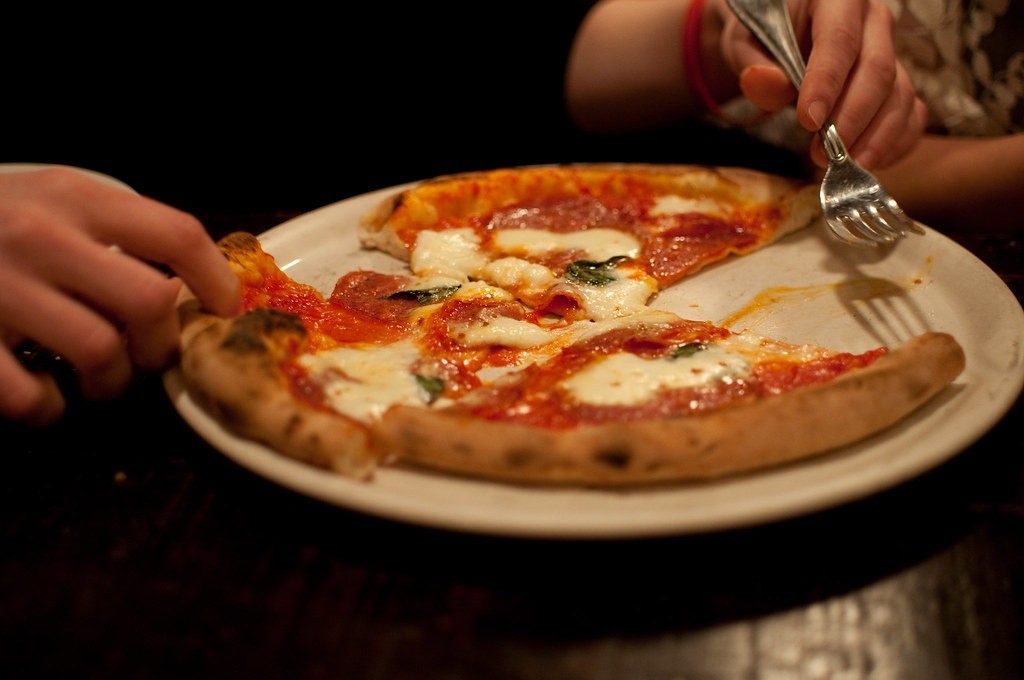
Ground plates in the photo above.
[161,180,1024,539]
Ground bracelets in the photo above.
[681,0,724,116]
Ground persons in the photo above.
[0,0,925,420]
[873,0,1024,214]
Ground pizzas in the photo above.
[356,164,825,321]
[378,306,968,484]
[176,231,543,480]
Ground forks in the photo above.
[725,0,926,250]
